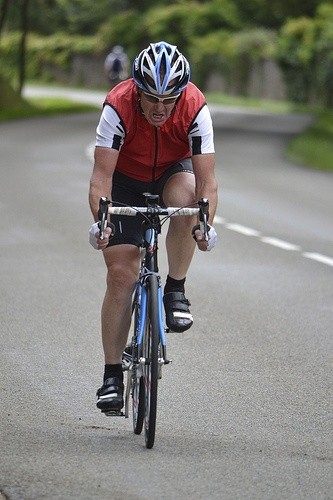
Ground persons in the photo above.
[89,41,218,413]
[105,44,131,92]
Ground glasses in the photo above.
[141,93,181,105]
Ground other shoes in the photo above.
[95,377,125,410]
[162,291,194,332]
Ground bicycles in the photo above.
[96,190,211,448]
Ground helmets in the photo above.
[132,40,191,97]
